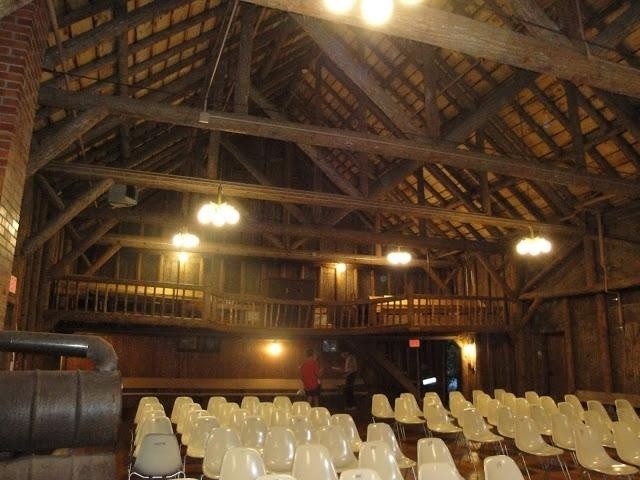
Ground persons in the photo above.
[340,349,357,395]
[298,349,321,407]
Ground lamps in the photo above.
[386,246,413,264]
[514,222,560,258]
[0,326,122,478]
[195,180,244,229]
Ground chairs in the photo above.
[131,384,640,477]
[0,326,122,478]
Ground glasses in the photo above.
[195,180,244,229]
[514,222,560,258]
[386,246,413,264]
